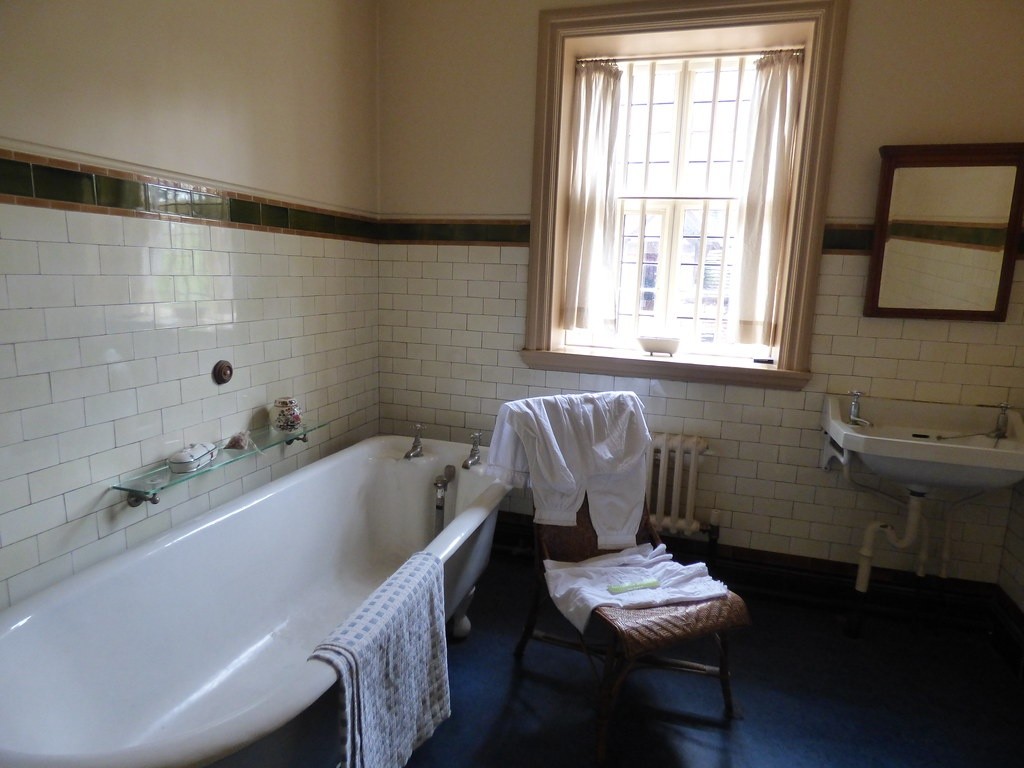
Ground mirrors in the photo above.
[863,142,1023,323]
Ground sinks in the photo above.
[819,393,1024,494]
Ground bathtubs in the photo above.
[1,434,516,768]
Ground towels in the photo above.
[305,550,452,768]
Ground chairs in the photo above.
[483,390,750,768]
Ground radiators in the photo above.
[642,431,723,558]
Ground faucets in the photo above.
[461,431,484,470]
[402,423,428,459]
[986,402,1014,440]
[848,390,874,429]
